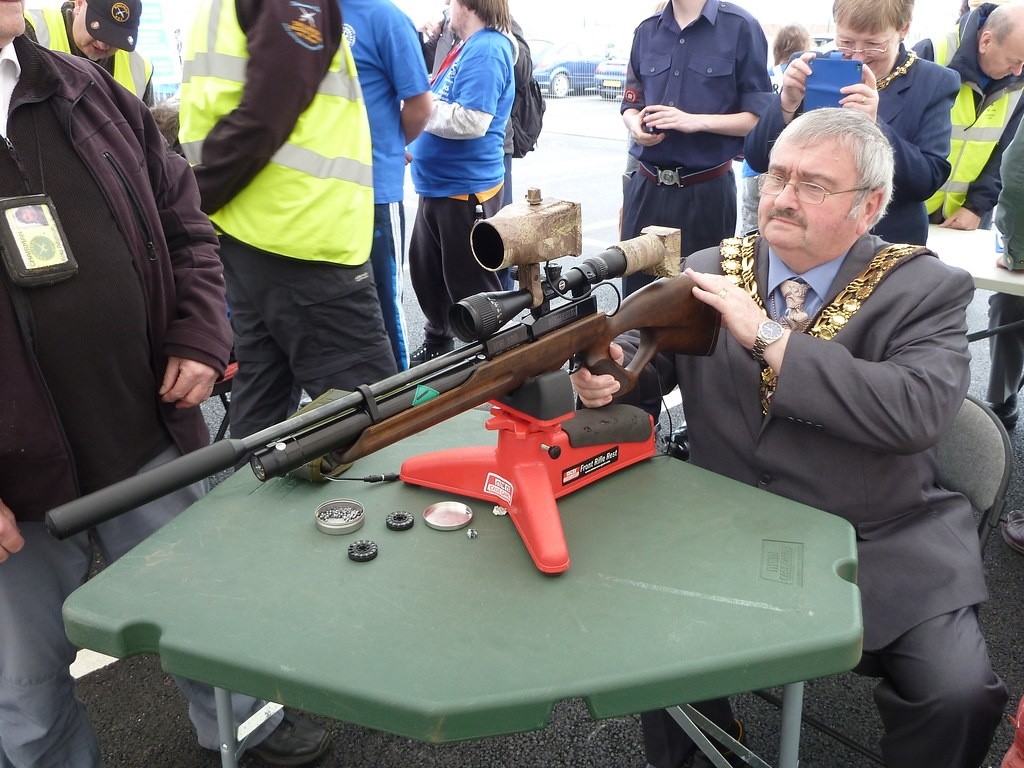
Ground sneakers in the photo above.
[409,336,455,367]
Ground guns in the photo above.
[41,187,721,543]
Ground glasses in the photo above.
[755,171,869,206]
[837,30,897,57]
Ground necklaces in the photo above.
[771,291,822,332]
[719,232,926,416]
[877,51,917,92]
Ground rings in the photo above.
[860,96,866,104]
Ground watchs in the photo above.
[752,321,785,367]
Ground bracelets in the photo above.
[781,102,801,113]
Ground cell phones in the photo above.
[804,58,863,113]
[646,112,663,133]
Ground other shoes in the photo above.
[250,710,330,766]
[1001,510,1024,554]
[664,421,689,459]
[984,392,1019,428]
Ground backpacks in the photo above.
[510,79,546,158]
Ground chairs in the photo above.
[751,393,1015,763]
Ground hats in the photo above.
[85,0,142,53]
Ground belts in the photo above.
[639,159,733,188]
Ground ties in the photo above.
[780,280,811,333]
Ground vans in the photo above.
[523,36,633,98]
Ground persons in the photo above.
[572,106,1012,768]
[741,0,961,247]
[911,1,1024,426]
[1001,508,1024,552]
[618,0,777,458]
[0,0,530,768]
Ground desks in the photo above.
[925,221,1024,343]
[61,383,863,768]
